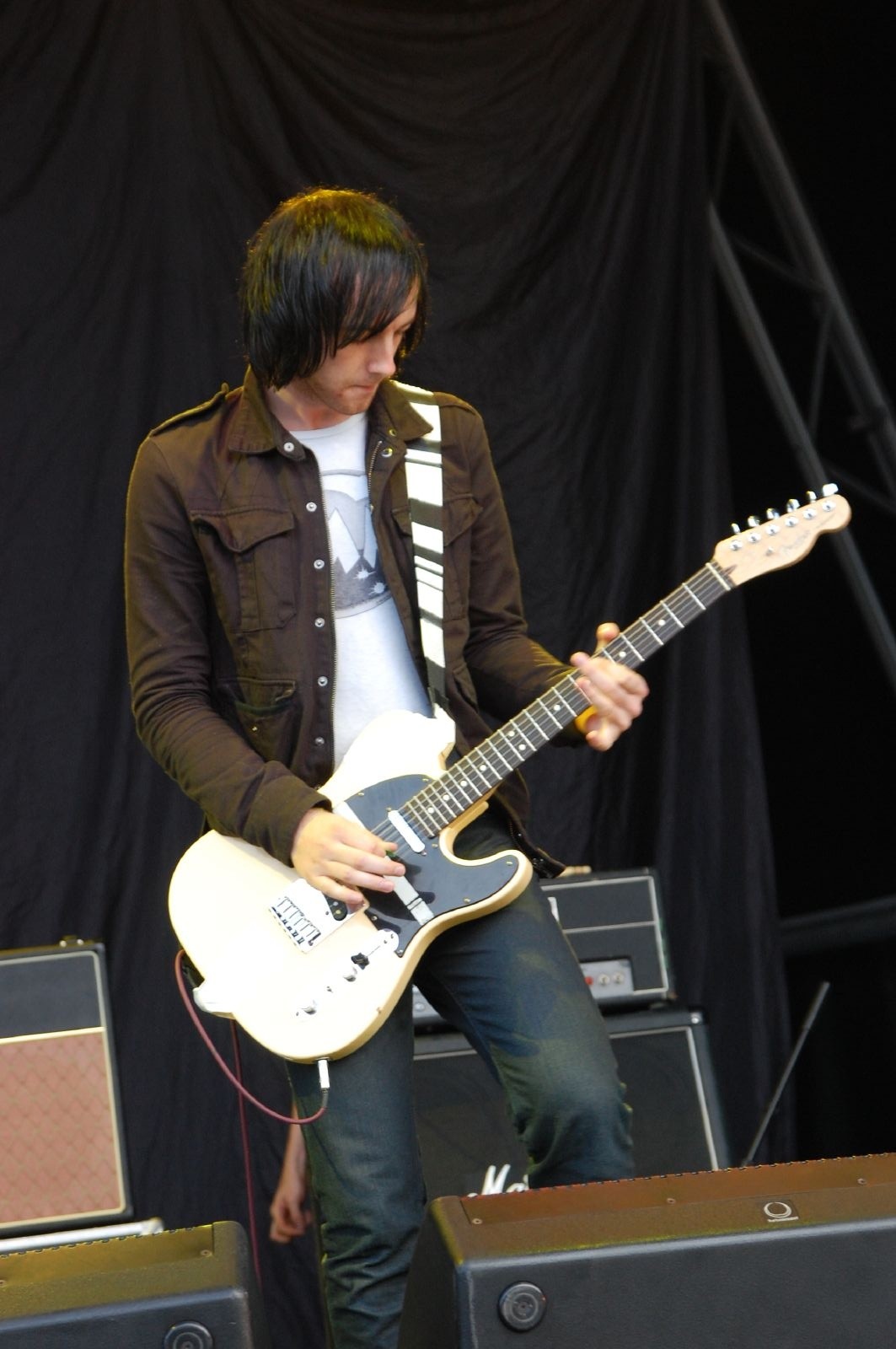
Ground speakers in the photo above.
[0,936,268,1349]
[402,864,896,1349]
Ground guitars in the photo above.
[166,481,855,1061]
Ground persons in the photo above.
[118,184,650,1349]
